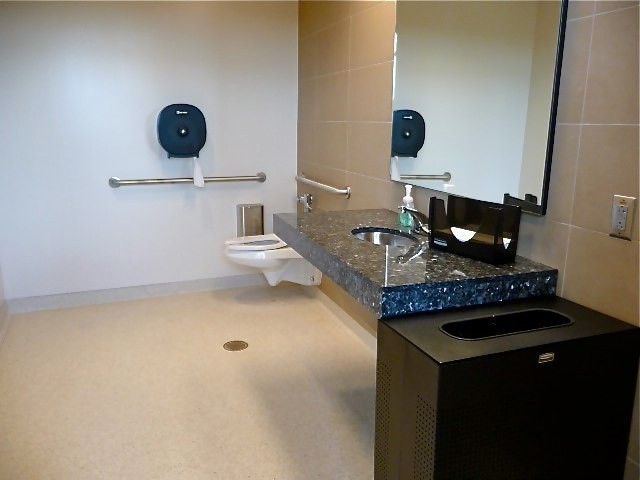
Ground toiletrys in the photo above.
[399,184,417,228]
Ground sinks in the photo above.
[351,226,417,251]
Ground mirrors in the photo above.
[389,0,569,215]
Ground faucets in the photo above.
[398,203,430,235]
[396,237,433,265]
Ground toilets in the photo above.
[222,233,323,288]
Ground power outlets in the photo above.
[609,194,637,241]
